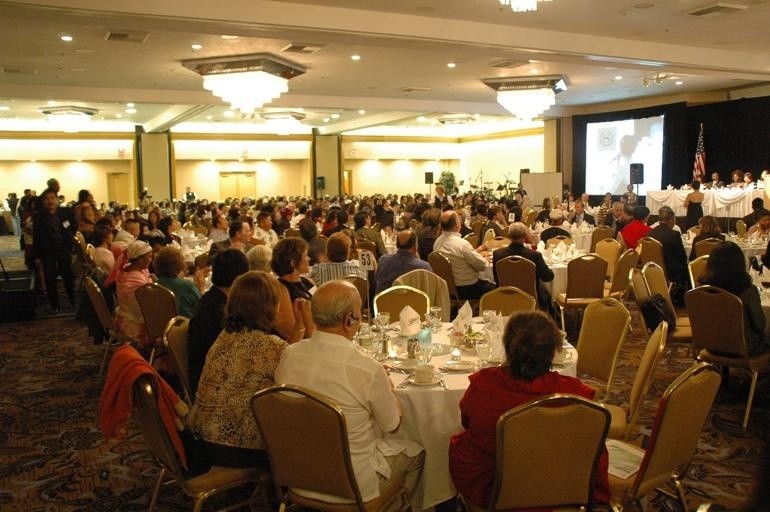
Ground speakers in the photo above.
[425,172,433,184]
[630,163,643,184]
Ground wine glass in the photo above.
[681,226,769,249]
[750,270,770,305]
[529,219,597,236]
[665,178,766,191]
[558,198,602,215]
[477,240,580,266]
[174,225,214,298]
[354,304,572,388]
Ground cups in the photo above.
[347,225,398,249]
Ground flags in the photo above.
[693,126,708,182]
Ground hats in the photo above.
[125,240,153,260]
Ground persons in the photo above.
[1,167,769,511]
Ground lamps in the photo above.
[37,105,100,134]
[180,51,307,115]
[477,74,569,123]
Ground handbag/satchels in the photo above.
[639,293,676,333]
